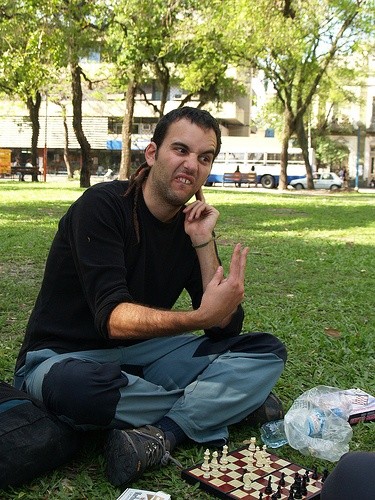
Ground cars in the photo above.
[290,172,343,191]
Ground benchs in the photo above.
[11,166,41,182]
[222,172,258,188]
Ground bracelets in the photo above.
[192,235,222,248]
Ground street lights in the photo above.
[355,120,363,192]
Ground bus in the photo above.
[204,136,315,190]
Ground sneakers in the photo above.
[96,426,183,486]
[241,392,284,424]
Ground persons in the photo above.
[234,166,242,187]
[343,167,348,187]
[247,166,256,188]
[13,106,288,487]
[96,163,104,177]
[14,156,39,183]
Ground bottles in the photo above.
[261,408,341,449]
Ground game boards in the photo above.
[181,443,324,500]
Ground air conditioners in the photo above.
[142,124,150,130]
[174,94,188,100]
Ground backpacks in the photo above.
[0,380,79,487]
